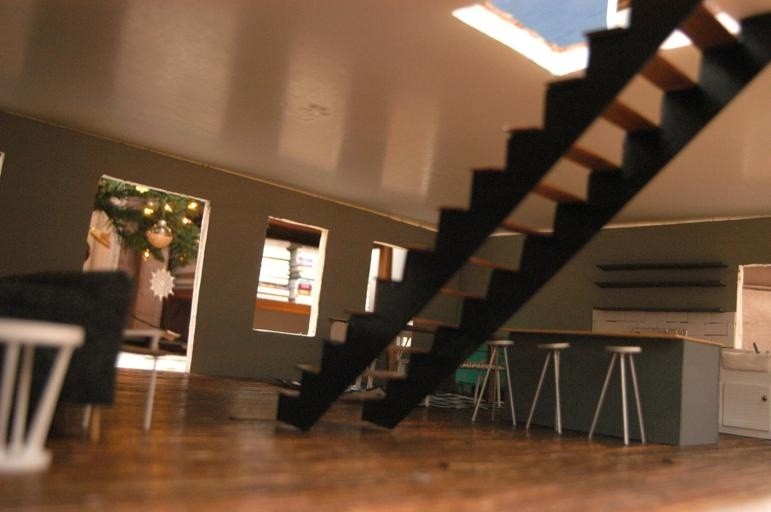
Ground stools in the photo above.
[518,343,578,434]
[585,345,649,446]
[466,338,515,424]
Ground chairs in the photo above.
[0,268,134,440]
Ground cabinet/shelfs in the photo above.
[592,256,726,314]
[719,379,769,433]
